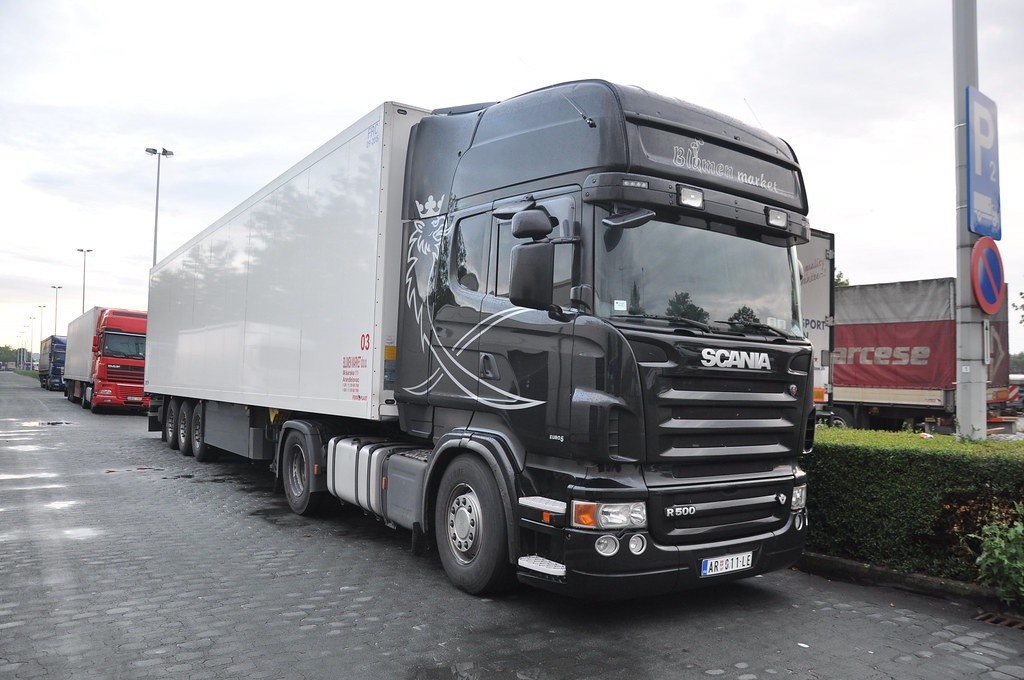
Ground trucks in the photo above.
[798,228,835,421]
[176,322,312,395]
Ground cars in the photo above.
[34,362,39,371]
[22,362,34,371]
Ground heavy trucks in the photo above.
[144,80,815,599]
[63,306,151,414]
[39,335,67,391]
[832,278,1010,436]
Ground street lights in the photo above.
[51,286,62,335]
[39,306,46,340]
[77,249,93,314]
[30,317,36,370]
[17,326,33,371]
[145,147,173,266]
